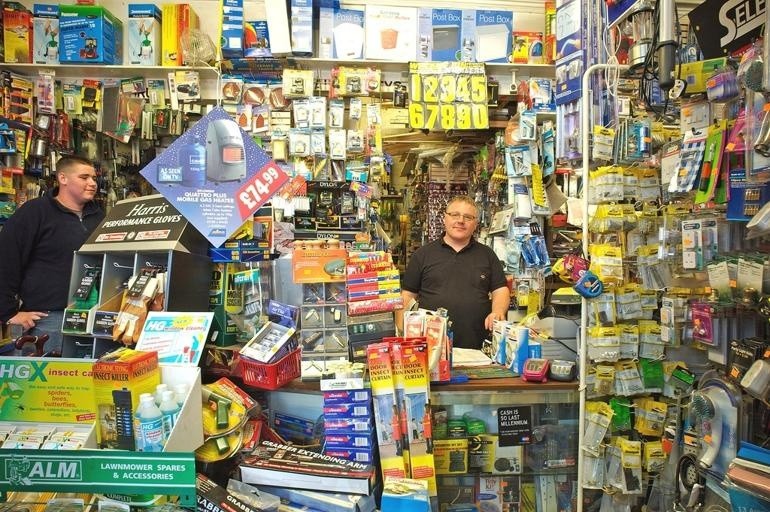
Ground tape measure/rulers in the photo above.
[573,269,604,298]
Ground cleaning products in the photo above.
[135,384,179,451]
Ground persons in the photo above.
[401,194,512,350]
[1,153,107,360]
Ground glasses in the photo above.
[446,212,475,222]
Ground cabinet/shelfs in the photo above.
[421,350,585,512]
